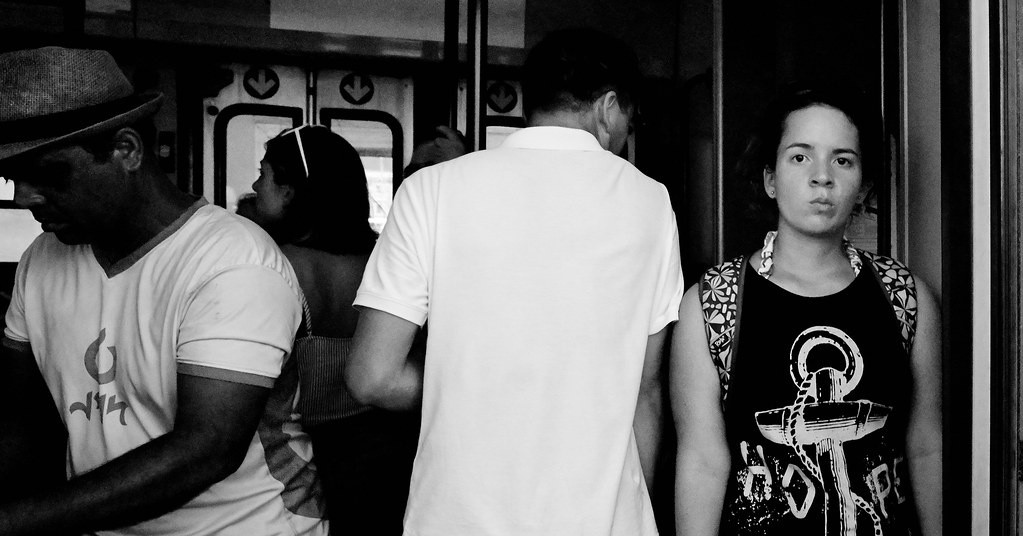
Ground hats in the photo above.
[0,46,164,164]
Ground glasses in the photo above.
[280,121,327,176]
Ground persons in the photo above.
[345,23,686,536]
[228,119,465,536]
[670,88,946,536]
[0,47,330,536]
